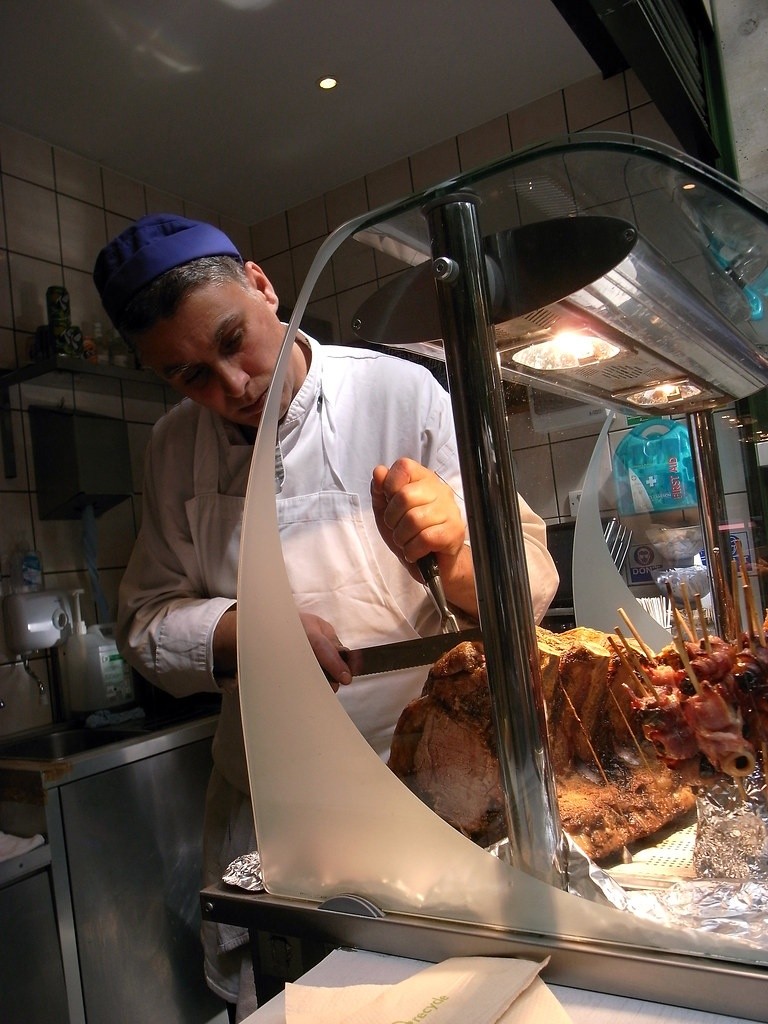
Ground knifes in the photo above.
[322,628,483,683]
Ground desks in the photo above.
[238,948,768,1024]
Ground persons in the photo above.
[92,210,558,1024]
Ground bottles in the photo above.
[92,321,109,365]
[110,328,129,368]
[87,625,135,710]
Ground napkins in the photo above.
[283,956,573,1024]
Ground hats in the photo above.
[92,214,244,326]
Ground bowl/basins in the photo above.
[645,526,703,560]
[652,565,709,604]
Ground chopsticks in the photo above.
[635,597,673,628]
